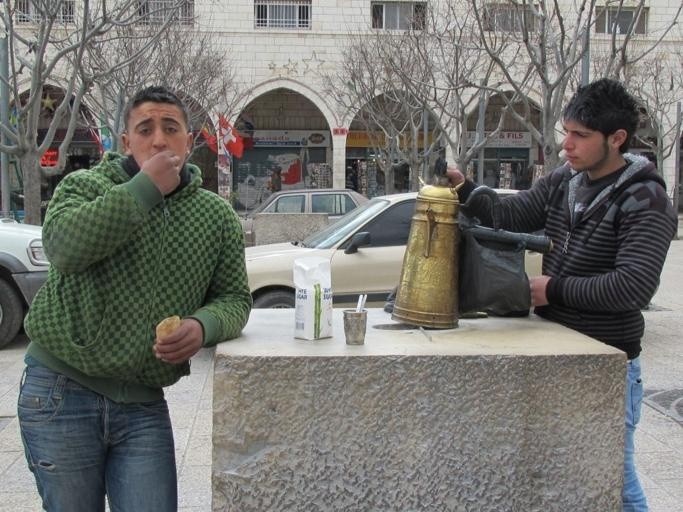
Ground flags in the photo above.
[202,127,217,153]
[98,113,112,149]
[220,114,244,159]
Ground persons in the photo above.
[18,85,254,511]
[446,77,678,512]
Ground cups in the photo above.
[343,309,368,345]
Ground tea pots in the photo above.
[391,173,461,329]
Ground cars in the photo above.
[236,186,370,246]
[0,217,51,348]
[243,189,544,309]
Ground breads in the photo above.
[156,314,180,339]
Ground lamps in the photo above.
[278,104,286,121]
